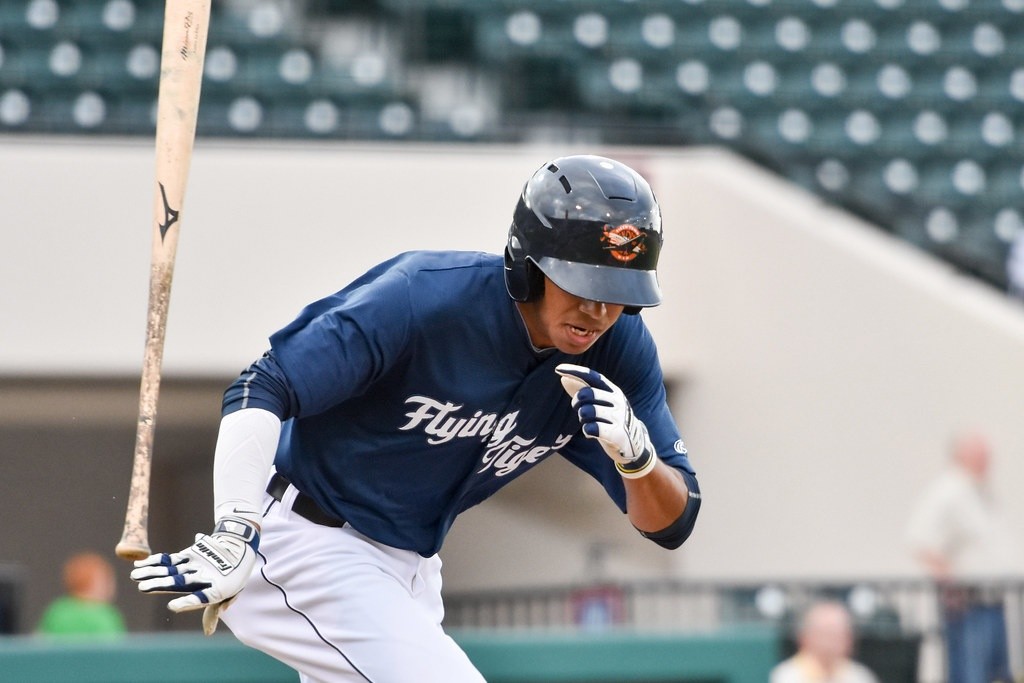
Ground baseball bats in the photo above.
[112,1,213,561]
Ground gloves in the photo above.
[130,517,259,636]
[555,363,656,479]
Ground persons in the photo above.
[565,539,628,629]
[918,426,1018,683]
[128,155,700,683]
[33,550,127,633]
[766,596,879,683]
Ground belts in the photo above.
[265,473,345,527]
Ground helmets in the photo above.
[503,155,665,317]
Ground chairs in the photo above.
[0,0,1024,288]
